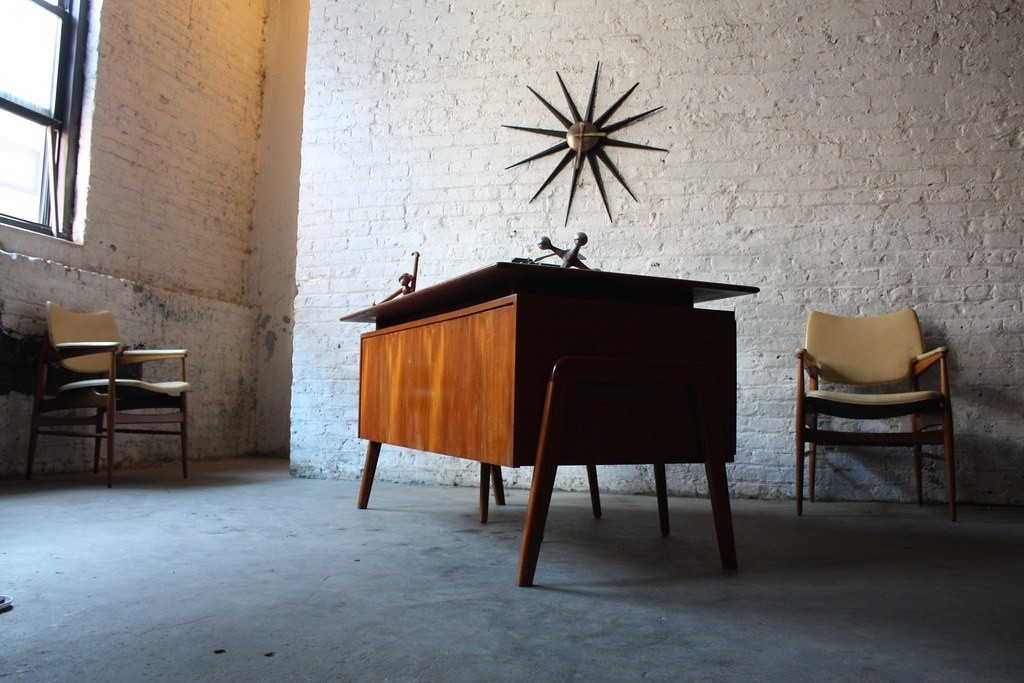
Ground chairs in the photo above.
[24,302,192,484]
[793,310,959,524]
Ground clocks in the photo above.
[499,60,669,227]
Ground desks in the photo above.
[337,262,760,586]
